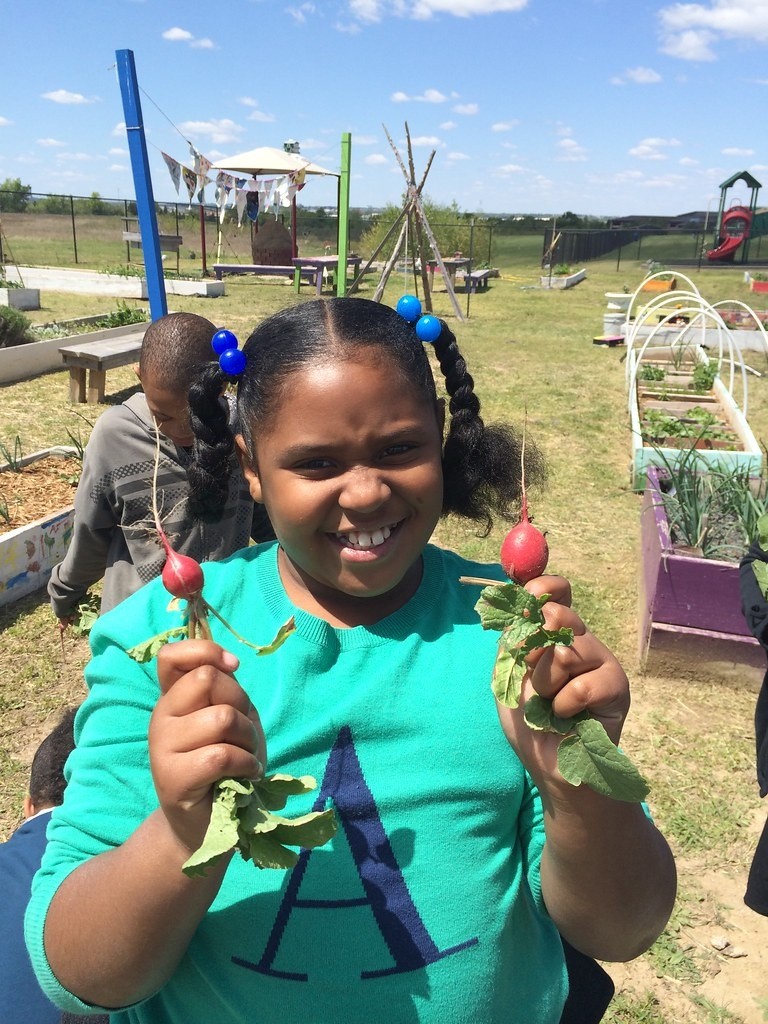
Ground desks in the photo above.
[425,257,474,293]
[291,255,364,296]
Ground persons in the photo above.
[48,311,277,627]
[0,709,76,1024]
[736,518,768,917]
[23,298,679,1024]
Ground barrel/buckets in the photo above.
[603,313,626,336]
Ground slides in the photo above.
[706,232,743,258]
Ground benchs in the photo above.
[212,262,318,288]
[463,269,490,295]
[58,322,225,406]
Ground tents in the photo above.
[207,146,340,282]
